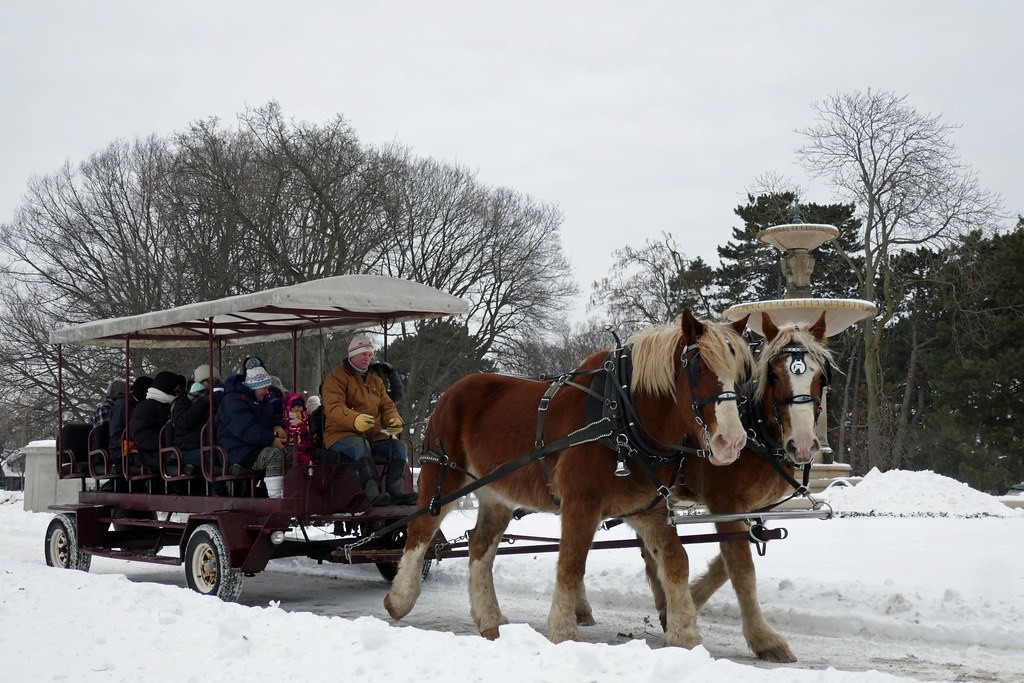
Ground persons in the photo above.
[320,332,419,507]
[90,358,288,499]
[304,394,327,468]
[287,395,321,476]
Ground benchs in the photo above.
[56,421,414,499]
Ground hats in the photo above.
[107,380,124,400]
[306,395,321,413]
[154,371,183,396]
[194,364,222,384]
[132,376,154,400]
[347,335,374,358]
[245,357,272,390]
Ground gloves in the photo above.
[387,418,403,433]
[355,414,375,432]
[382,362,392,374]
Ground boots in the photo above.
[354,457,391,507]
[386,459,418,506]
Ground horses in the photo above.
[385,308,845,663]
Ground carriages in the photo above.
[44,275,825,662]
